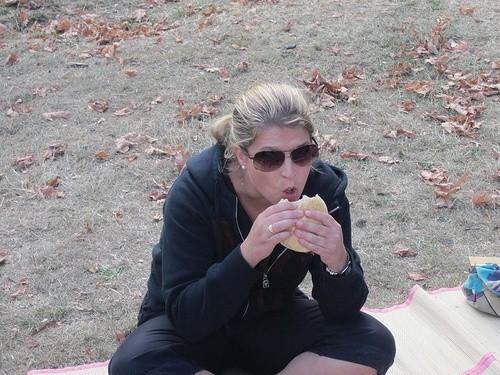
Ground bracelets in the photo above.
[326,249,351,277]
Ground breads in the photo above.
[279,195,328,253]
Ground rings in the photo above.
[269,223,275,234]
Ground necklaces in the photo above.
[238,170,268,212]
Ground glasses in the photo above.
[240,137,321,173]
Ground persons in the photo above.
[107,81,396,375]
